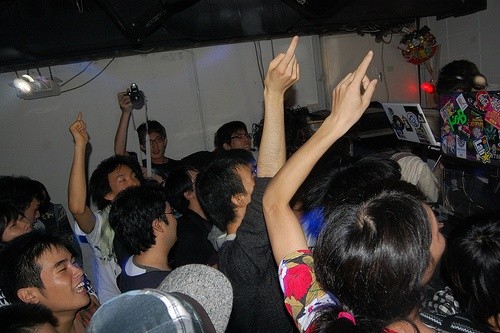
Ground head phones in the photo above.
[470,72,487,89]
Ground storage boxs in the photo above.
[351,128,393,154]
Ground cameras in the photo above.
[124,83,145,110]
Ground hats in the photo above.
[83,264,235,333]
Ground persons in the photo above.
[0,33,500,333]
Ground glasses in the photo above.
[230,133,252,140]
[150,137,164,145]
